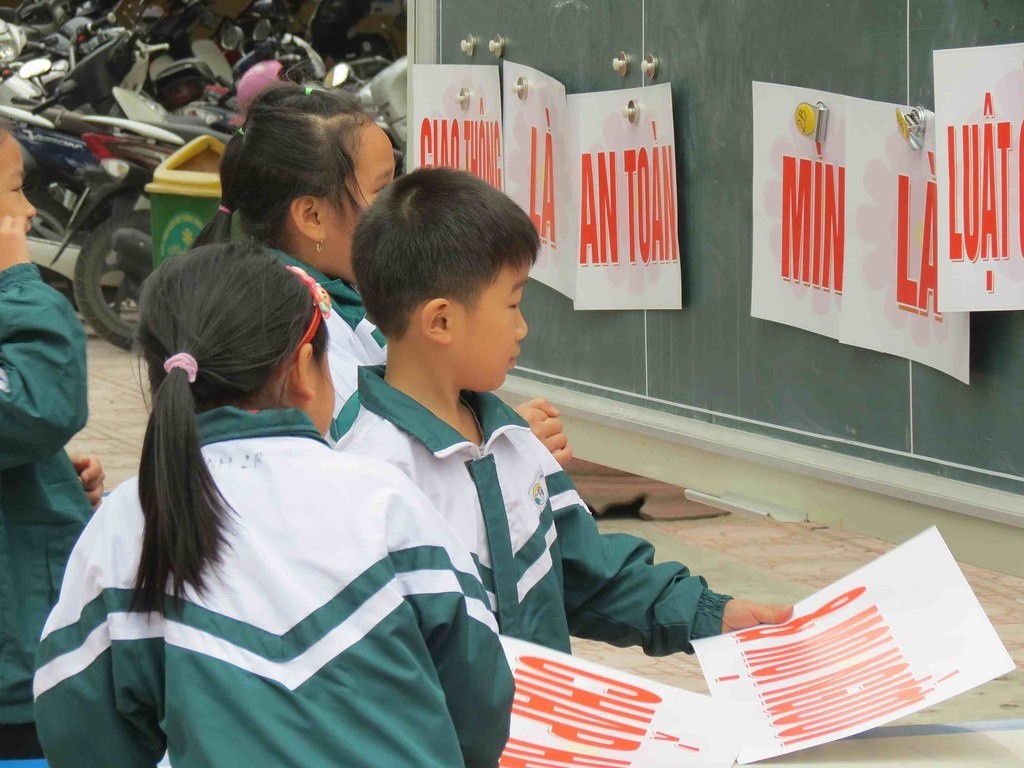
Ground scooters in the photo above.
[0,0,410,350]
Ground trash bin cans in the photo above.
[144,136,243,273]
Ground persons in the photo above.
[32,242,517,768]
[0,128,106,760]
[191,81,572,465]
[332,166,793,657]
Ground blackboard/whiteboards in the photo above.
[406,1,1024,530]
